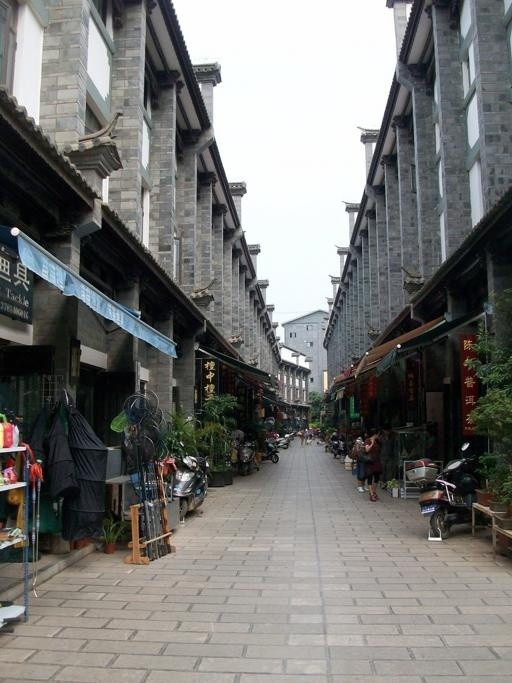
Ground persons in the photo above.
[298,426,320,446]
[351,426,393,502]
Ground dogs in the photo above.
[306,439,312,445]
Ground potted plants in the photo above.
[473,451,511,558]
[100,514,129,554]
[199,392,239,486]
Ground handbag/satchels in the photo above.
[349,444,356,459]
[359,448,374,464]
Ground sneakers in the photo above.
[371,492,379,501]
[357,486,369,492]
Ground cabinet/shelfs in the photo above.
[1,445,31,631]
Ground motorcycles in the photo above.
[166,440,211,521]
[227,429,294,476]
[405,442,487,539]
[330,432,350,459]
